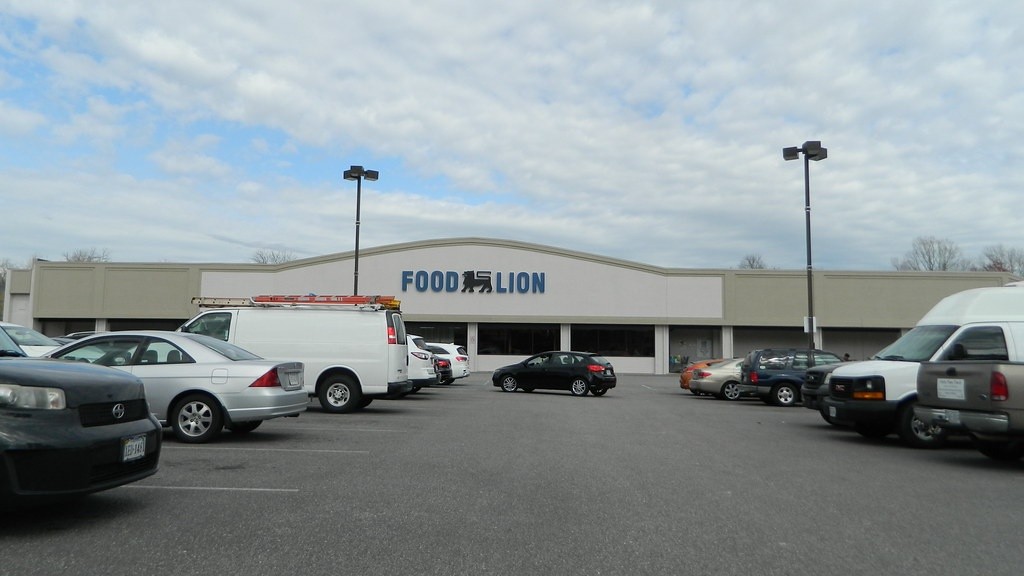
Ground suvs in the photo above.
[738,346,854,407]
[493,350,617,398]
[424,342,470,385]
[383,333,437,398]
[800,359,865,428]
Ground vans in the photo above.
[125,292,413,414]
[821,284,1023,448]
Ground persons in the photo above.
[843,353,850,360]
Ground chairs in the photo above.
[563,357,568,364]
[144,350,159,362]
[167,350,180,362]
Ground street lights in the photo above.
[783,138,830,350]
[343,164,380,296]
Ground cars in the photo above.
[36,329,310,446]
[689,357,747,402]
[407,351,455,395]
[0,321,62,357]
[50,337,105,364]
[679,360,727,395]
[0,322,164,518]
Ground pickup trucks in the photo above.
[911,358,1023,465]
